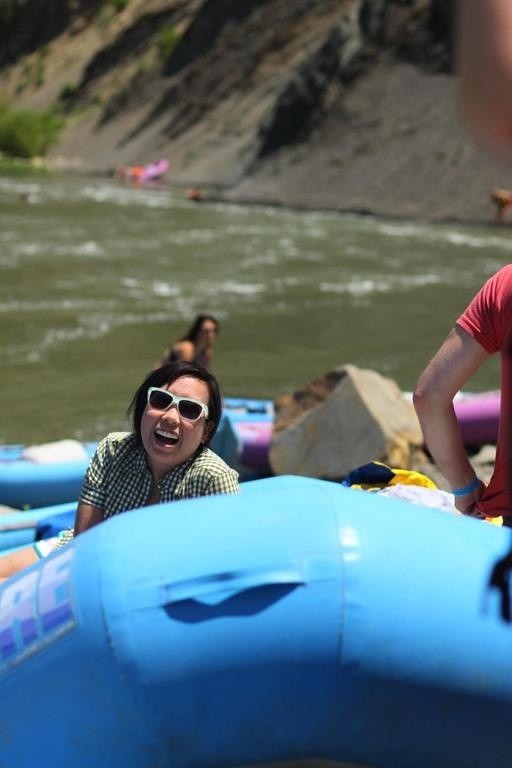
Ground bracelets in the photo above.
[447,476,481,501]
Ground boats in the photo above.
[1,398,511,768]
[127,158,169,182]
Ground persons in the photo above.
[147,311,223,378]
[1,356,242,592]
[413,262,512,534]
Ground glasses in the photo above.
[146,387,209,423]
